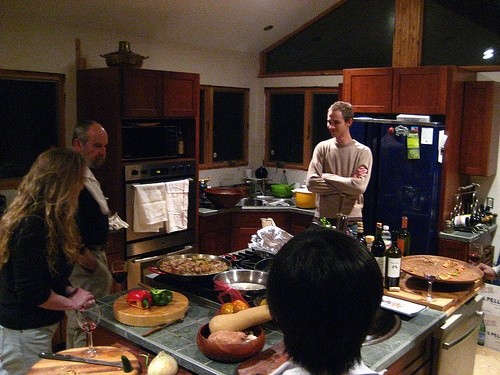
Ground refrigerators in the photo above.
[348,115,444,255]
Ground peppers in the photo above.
[121,355,132,372]
[126,288,172,310]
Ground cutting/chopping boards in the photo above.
[113,288,190,327]
[25,345,140,375]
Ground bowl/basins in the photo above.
[196,322,265,363]
[155,253,231,284]
[204,187,244,208]
[213,269,269,302]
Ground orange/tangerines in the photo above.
[220,300,268,314]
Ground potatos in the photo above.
[206,330,247,344]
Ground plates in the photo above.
[400,255,484,285]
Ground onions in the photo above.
[147,350,178,375]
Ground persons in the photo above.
[265,227,389,375]
[476,262,500,280]
[67,120,114,349]
[0,147,96,375]
[306,100,373,239]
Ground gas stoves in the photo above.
[147,247,280,331]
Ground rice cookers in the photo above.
[291,189,315,209]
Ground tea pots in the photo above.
[254,166,267,178]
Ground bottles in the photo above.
[200,181,207,200]
[245,167,252,177]
[443,220,454,234]
[300,179,306,188]
[336,216,410,289]
[467,205,493,227]
[280,170,288,184]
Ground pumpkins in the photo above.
[209,304,271,333]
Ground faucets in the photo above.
[246,178,267,196]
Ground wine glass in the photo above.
[469,242,484,266]
[76,303,101,358]
[421,264,438,303]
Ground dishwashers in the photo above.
[431,294,485,375]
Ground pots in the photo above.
[271,183,295,198]
[100,41,150,69]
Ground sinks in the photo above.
[242,196,296,208]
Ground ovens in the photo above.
[124,160,196,290]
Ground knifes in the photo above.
[38,351,123,367]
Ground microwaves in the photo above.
[121,118,196,161]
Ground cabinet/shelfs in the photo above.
[343,66,448,115]
[199,212,314,256]
[75,67,200,119]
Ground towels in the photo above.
[164,179,189,234]
[132,182,169,233]
